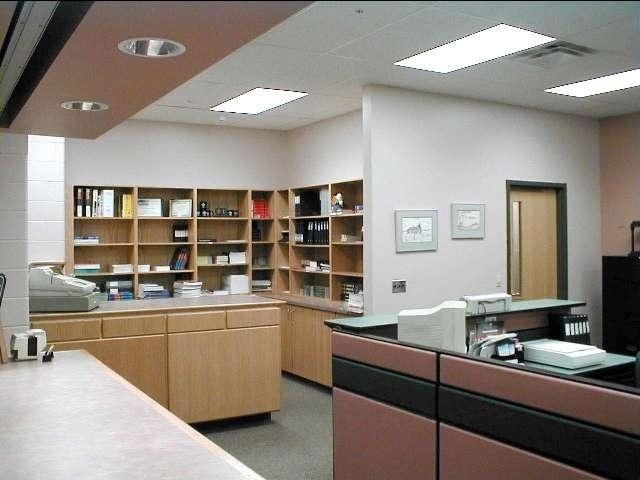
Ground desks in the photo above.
[0,349,269,480]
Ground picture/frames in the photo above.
[449,203,486,239]
[169,198,191,217]
[394,209,440,254]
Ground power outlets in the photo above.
[391,279,407,294]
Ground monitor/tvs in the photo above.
[397,301,467,354]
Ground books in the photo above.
[74,187,132,244]
[252,199,270,219]
[74,247,190,273]
[519,338,607,369]
[252,280,272,291]
[97,274,249,300]
[197,239,246,265]
[294,188,363,309]
[548,312,590,345]
[173,223,188,242]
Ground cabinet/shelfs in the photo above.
[65,182,277,297]
[277,177,363,307]
[600,253,640,355]
[166,305,280,425]
[276,300,362,388]
[31,313,169,412]
